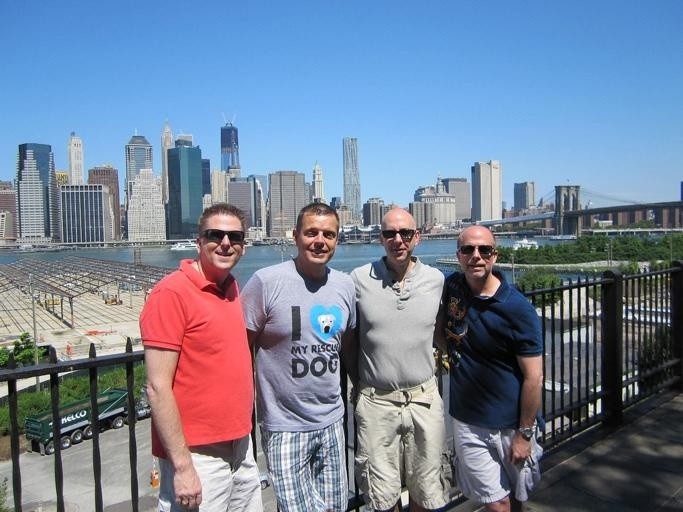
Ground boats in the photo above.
[13,244,82,252]
[170,242,198,251]
[549,234,578,240]
[511,235,539,251]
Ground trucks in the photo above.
[24,383,152,456]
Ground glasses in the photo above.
[456,245,494,257]
[199,229,247,244]
[381,229,418,241]
